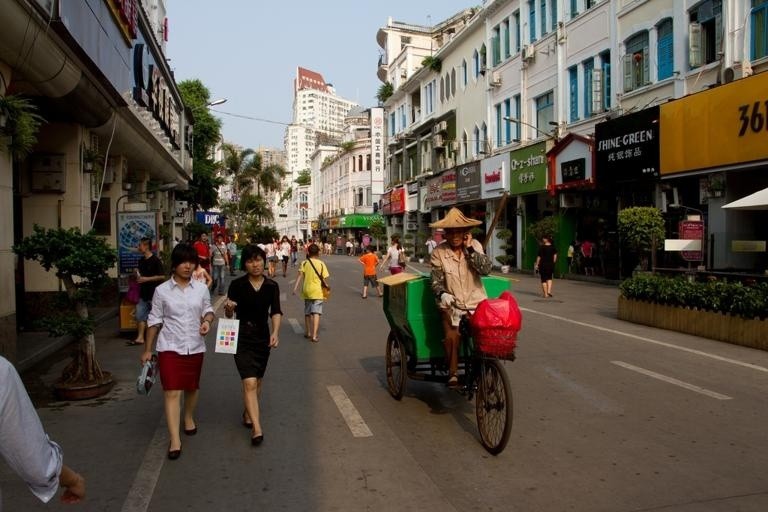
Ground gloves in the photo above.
[441,292,455,308]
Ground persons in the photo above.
[379,234,405,276]
[535,237,557,299]
[125,236,166,345]
[425,235,436,256]
[296,232,370,254]
[222,242,281,446]
[188,222,296,297]
[357,246,382,299]
[0,352,86,505]
[564,237,609,275]
[428,207,495,389]
[291,243,331,343]
[140,243,214,462]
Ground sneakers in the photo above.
[448,371,461,390]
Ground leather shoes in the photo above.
[169,436,183,459]
[184,422,196,435]
[243,408,254,429]
[251,435,263,446]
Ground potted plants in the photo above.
[402,234,414,263]
[124,176,136,189]
[0,94,49,163]
[495,230,514,274]
[146,180,162,198]
[417,232,427,263]
[10,221,120,401]
[82,146,105,171]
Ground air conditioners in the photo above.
[433,121,458,152]
[521,44,534,62]
[488,71,500,85]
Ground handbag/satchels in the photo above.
[321,285,331,302]
[136,352,159,396]
[398,251,405,265]
[127,275,140,304]
[215,317,240,355]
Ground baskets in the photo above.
[468,323,521,362]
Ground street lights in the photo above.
[192,98,228,117]
[114,182,180,288]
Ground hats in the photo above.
[428,207,483,229]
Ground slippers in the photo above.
[126,340,145,345]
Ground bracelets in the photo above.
[202,319,210,325]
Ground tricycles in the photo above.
[378,271,520,456]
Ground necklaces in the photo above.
[248,277,264,292]
